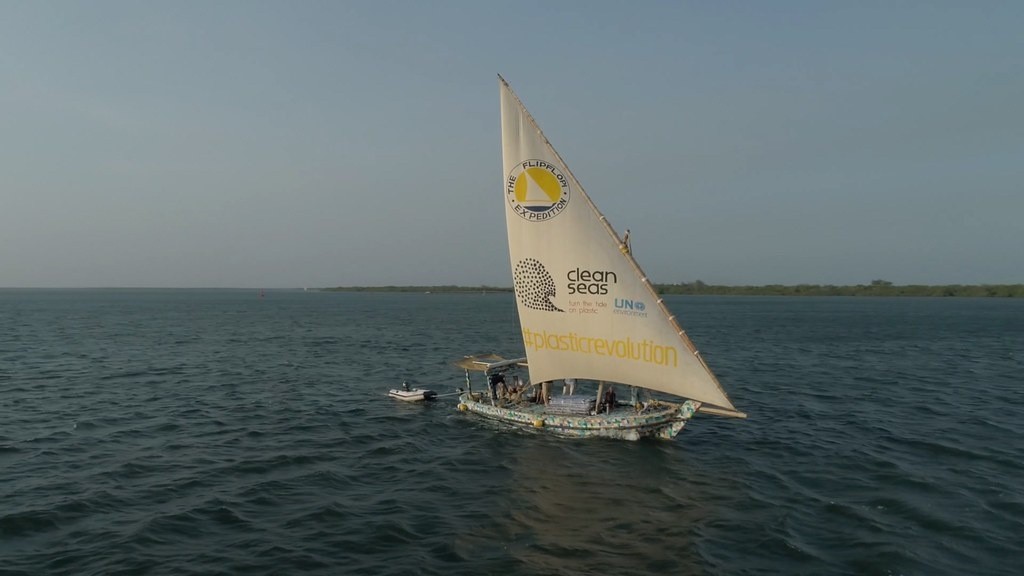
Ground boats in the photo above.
[389,380,437,402]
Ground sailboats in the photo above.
[457,72,747,441]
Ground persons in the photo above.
[629,385,641,412]
[605,386,616,411]
[561,379,578,397]
[536,380,553,404]
[491,372,509,400]
